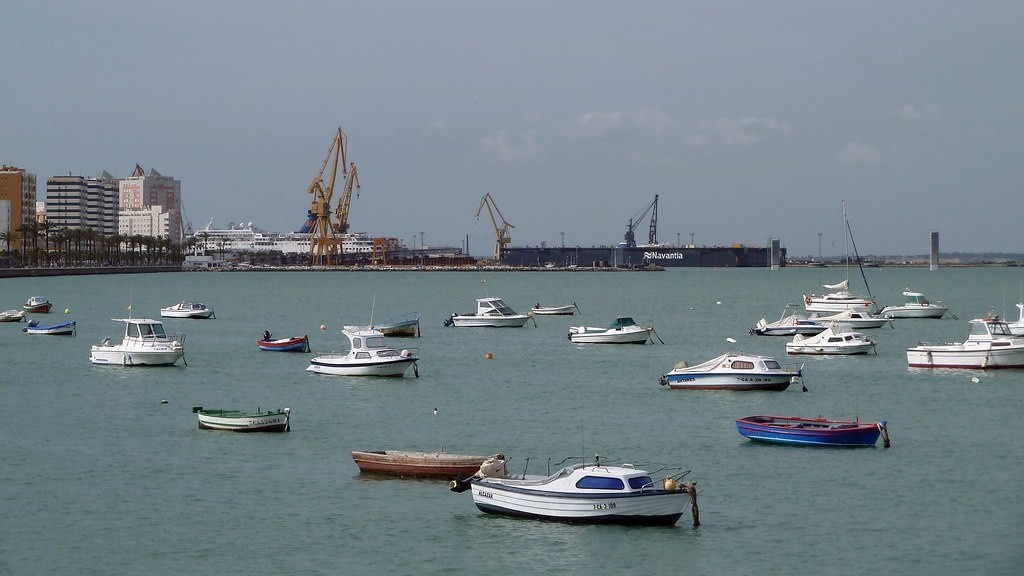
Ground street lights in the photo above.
[816,231,823,267]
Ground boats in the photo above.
[258,330,311,352]
[531,302,583,317]
[22,296,53,313]
[568,315,655,345]
[191,406,292,433]
[351,448,508,480]
[746,303,828,337]
[449,457,700,530]
[160,300,216,319]
[88,317,188,368]
[905,307,1024,370]
[809,306,895,330]
[734,413,892,449]
[304,291,420,378]
[785,322,879,358]
[658,350,811,392]
[967,301,1024,338]
[442,275,538,329]
[879,287,950,318]
[343,311,423,338]
[0,310,27,322]
[21,318,77,336]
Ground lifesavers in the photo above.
[805,296,811,305]
[921,300,929,307]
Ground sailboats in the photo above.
[798,200,880,315]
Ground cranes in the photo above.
[624,193,660,248]
[475,192,515,264]
[302,125,361,266]
